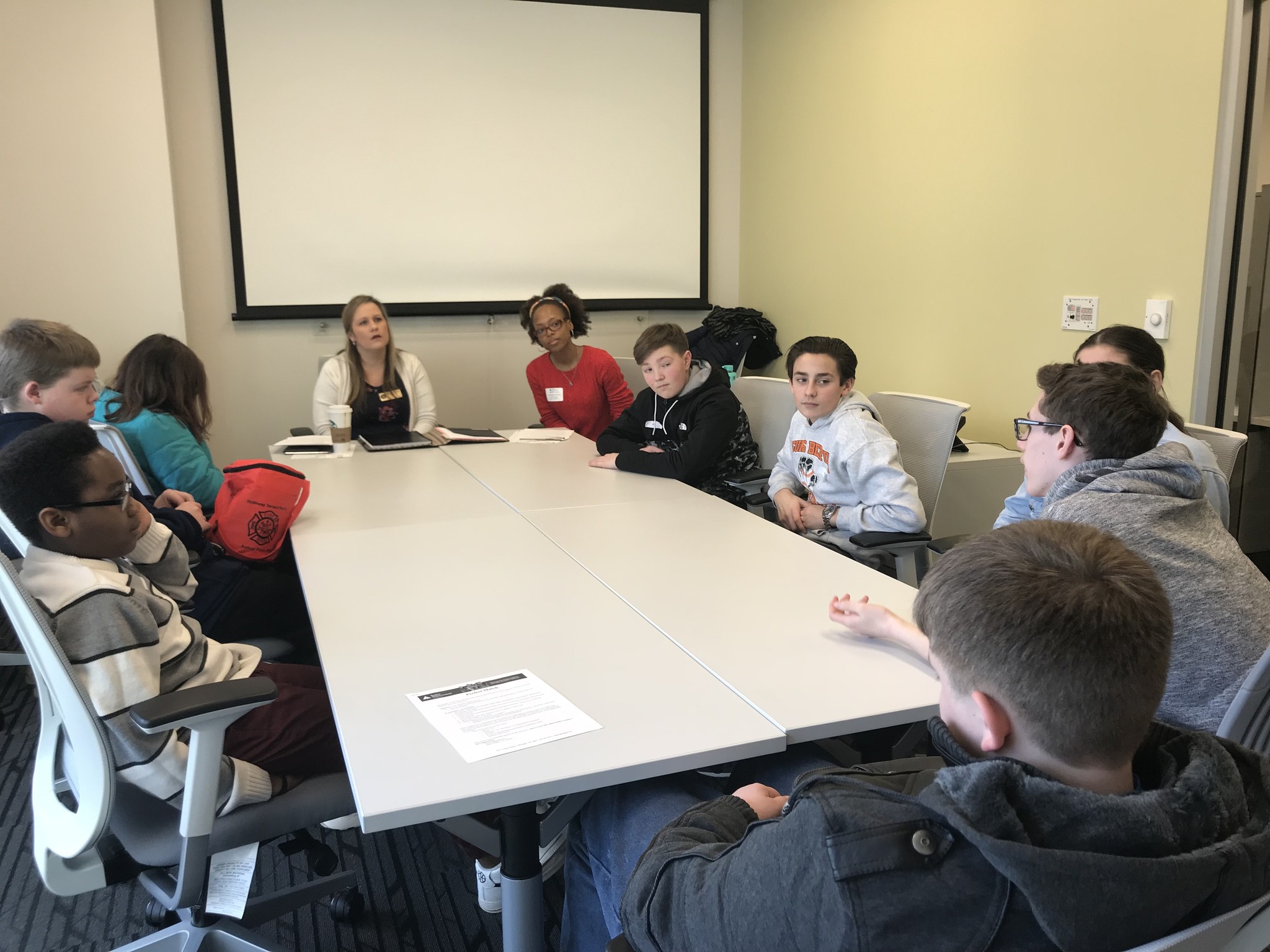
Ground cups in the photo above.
[328,405,353,454]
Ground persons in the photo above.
[589,323,762,512]
[991,325,1270,734]
[767,336,927,578]
[1,318,572,913]
[312,296,436,437]
[519,284,635,441]
[560,518,1270,952]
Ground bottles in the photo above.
[722,364,737,387]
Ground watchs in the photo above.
[822,505,840,531]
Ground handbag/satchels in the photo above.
[205,459,310,560]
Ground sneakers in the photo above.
[475,824,568,913]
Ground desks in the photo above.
[269,446,786,952]
[437,426,941,746]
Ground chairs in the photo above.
[613,353,1270,952]
[0,510,365,952]
[88,420,156,497]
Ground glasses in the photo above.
[48,474,132,511]
[1014,418,1083,447]
[533,318,568,338]
